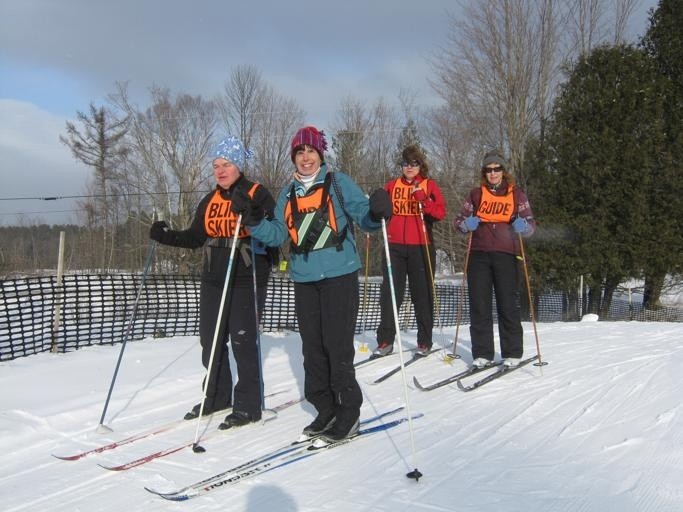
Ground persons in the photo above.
[148,134,276,431]
[371,144,447,357]
[452,149,537,370]
[229,125,392,443]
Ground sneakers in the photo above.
[302,412,337,437]
[319,415,361,444]
[183,398,232,421]
[415,342,432,357]
[369,340,394,360]
[472,356,493,369]
[218,411,262,432]
[500,355,520,369]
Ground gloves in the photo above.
[150,222,169,242]
[410,187,426,203]
[369,188,393,219]
[459,216,481,233]
[511,216,532,235]
[231,189,263,226]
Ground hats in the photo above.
[212,134,245,173]
[483,150,506,170]
[291,125,328,160]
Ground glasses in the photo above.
[484,167,503,173]
[399,160,420,167]
